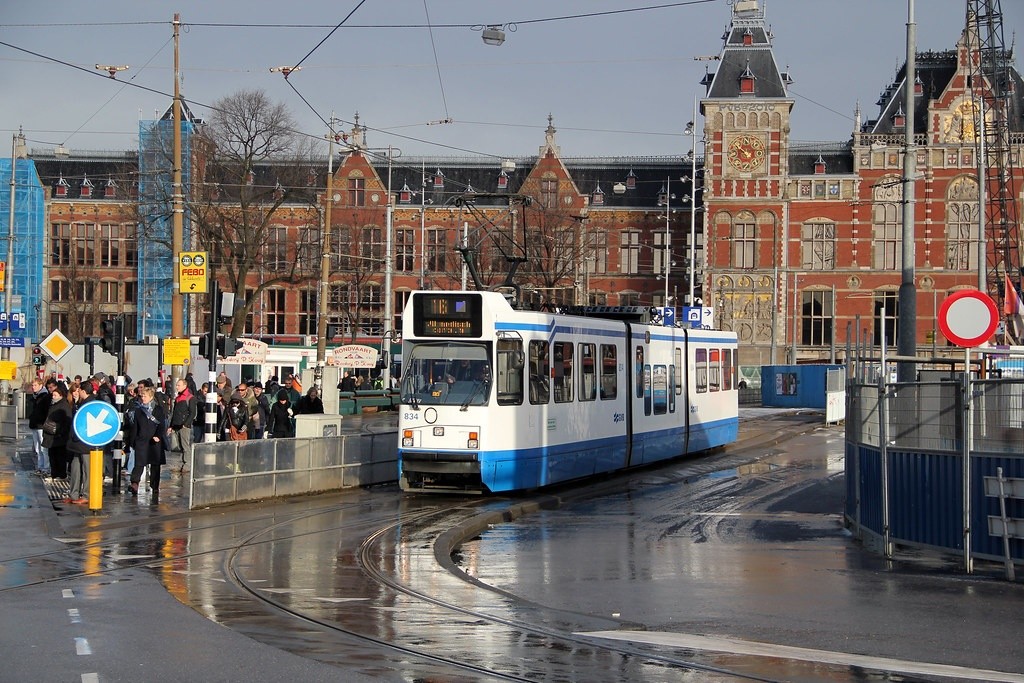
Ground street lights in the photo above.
[657,194,677,308]
[413,176,432,289]
[316,250,332,387]
[683,120,711,326]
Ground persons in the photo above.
[390,374,397,388]
[46,379,58,396]
[167,378,196,473]
[41,389,72,481]
[69,375,82,392]
[93,373,117,487]
[50,371,56,380]
[125,378,165,504]
[28,379,50,479]
[194,372,325,442]
[374,377,383,389]
[338,371,354,391]
[64,381,97,504]
[123,371,133,389]
[184,372,197,394]
[165,376,172,411]
[68,384,84,408]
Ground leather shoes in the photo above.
[62,498,89,504]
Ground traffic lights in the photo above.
[31,346,43,366]
[99,320,124,355]
[220,291,247,326]
[220,335,243,359]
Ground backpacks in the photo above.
[186,394,197,420]
[375,380,382,390]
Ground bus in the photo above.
[377,289,739,494]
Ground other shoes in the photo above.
[45,477,65,482]
[128,485,138,497]
[30,470,51,478]
[152,489,160,505]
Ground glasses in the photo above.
[237,389,246,391]
[285,383,291,385]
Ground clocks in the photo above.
[727,133,765,172]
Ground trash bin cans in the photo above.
[12,390,26,420]
[26,394,34,417]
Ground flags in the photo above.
[1005,272,1024,338]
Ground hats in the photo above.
[277,390,288,401]
[216,376,226,384]
[253,382,263,390]
[230,393,243,401]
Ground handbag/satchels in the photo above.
[230,424,247,441]
[163,434,171,452]
[42,417,61,435]
